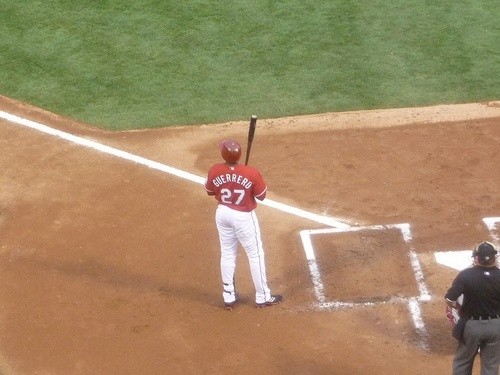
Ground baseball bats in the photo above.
[244,114,258,166]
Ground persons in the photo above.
[203,140,283,311]
[444,240,500,375]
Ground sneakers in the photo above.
[256,295,282,307]
[224,293,239,308]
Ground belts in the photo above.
[468,315,499,320]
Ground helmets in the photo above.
[470,241,498,267]
[221,140,242,163]
